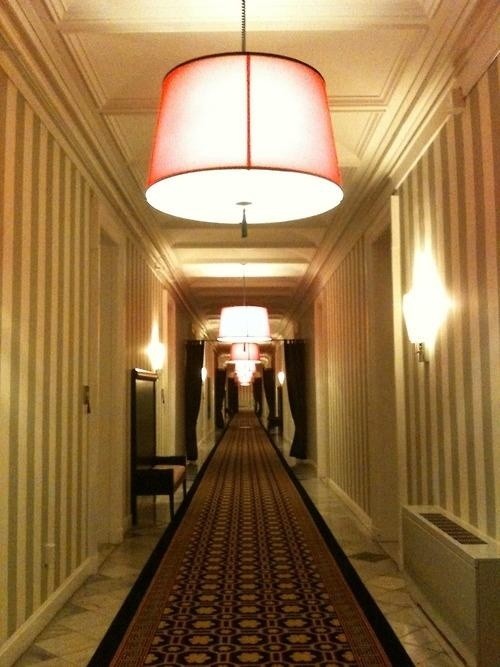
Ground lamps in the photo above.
[218,261,269,387]
[401,289,432,363]
[144,0,344,239]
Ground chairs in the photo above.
[130,367,186,524]
